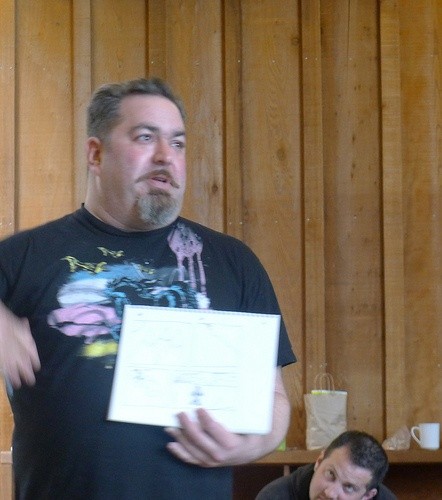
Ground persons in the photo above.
[0,77,298,500]
[256,430,398,500]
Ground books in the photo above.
[107,304,281,434]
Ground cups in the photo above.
[411,422,441,451]
